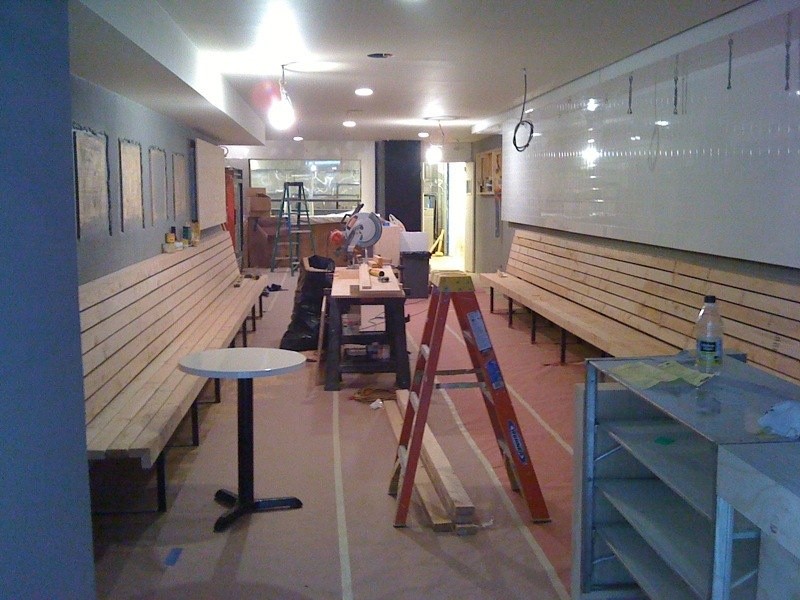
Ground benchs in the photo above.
[480,228,800,390]
[81,229,270,517]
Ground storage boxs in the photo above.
[245,193,271,212]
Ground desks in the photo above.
[324,264,411,391]
[249,216,289,268]
[179,347,307,534]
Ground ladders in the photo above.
[271,182,316,276]
[388,271,554,530]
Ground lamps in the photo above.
[267,65,295,131]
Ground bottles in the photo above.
[182,225,191,241]
[694,295,724,377]
[171,226,177,241]
[167,233,175,243]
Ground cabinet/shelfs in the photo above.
[579,349,800,600]
